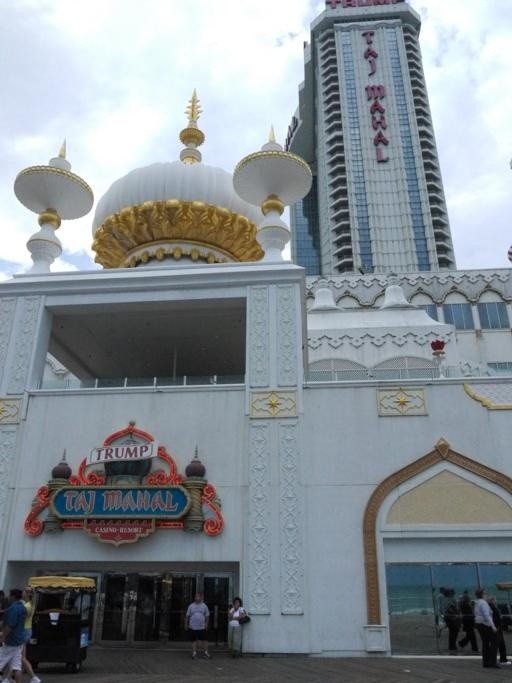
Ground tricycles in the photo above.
[22,578,94,675]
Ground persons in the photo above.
[487,595,512,665]
[184,591,211,659]
[474,588,502,669]
[0,587,42,683]
[226,597,246,658]
[435,587,479,655]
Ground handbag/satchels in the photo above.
[239,615,249,624]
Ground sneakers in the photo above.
[31,676,41,683]
[191,652,210,658]
[482,661,511,668]
